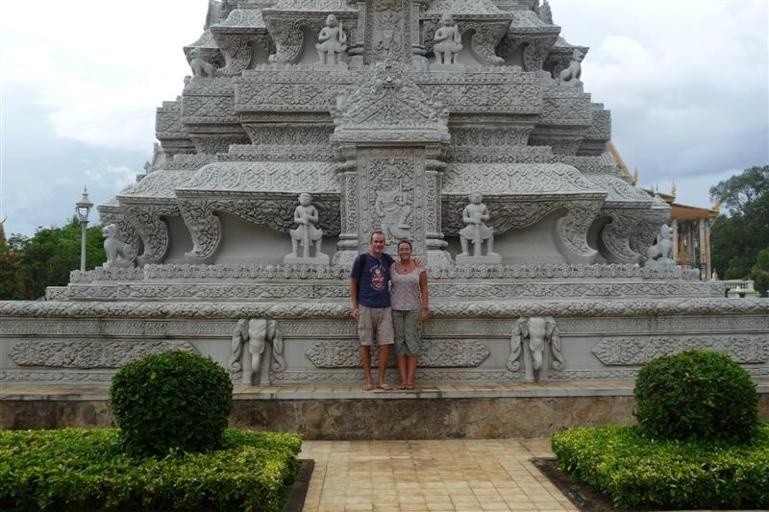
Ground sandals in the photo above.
[399,382,415,388]
[380,385,392,390]
[363,384,372,389]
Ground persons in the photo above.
[350,231,428,390]
[433,12,462,66]
[315,14,347,65]
[288,193,326,258]
[457,190,499,257]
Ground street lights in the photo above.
[74,184,94,271]
